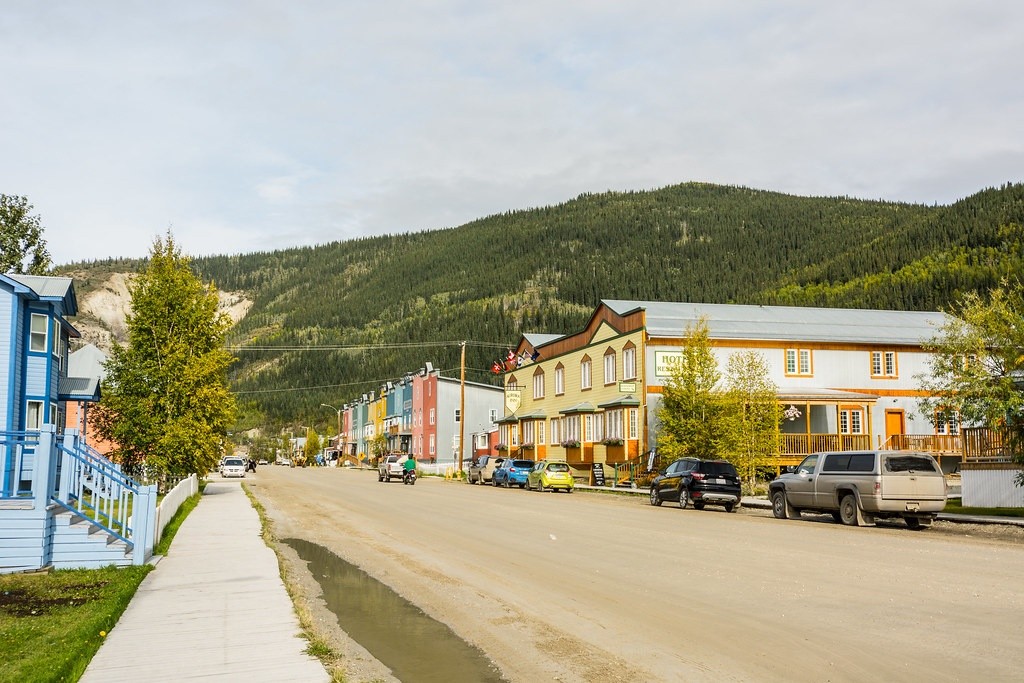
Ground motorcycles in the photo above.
[399,463,417,485]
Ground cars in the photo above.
[259,459,267,465]
[526,463,574,492]
[221,458,245,477]
[491,459,535,487]
[274,457,295,466]
[468,455,507,485]
[218,455,256,473]
[378,456,405,482]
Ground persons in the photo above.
[403,454,415,472]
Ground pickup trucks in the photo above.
[768,449,948,523]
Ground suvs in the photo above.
[649,457,742,513]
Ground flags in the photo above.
[491,348,541,374]
